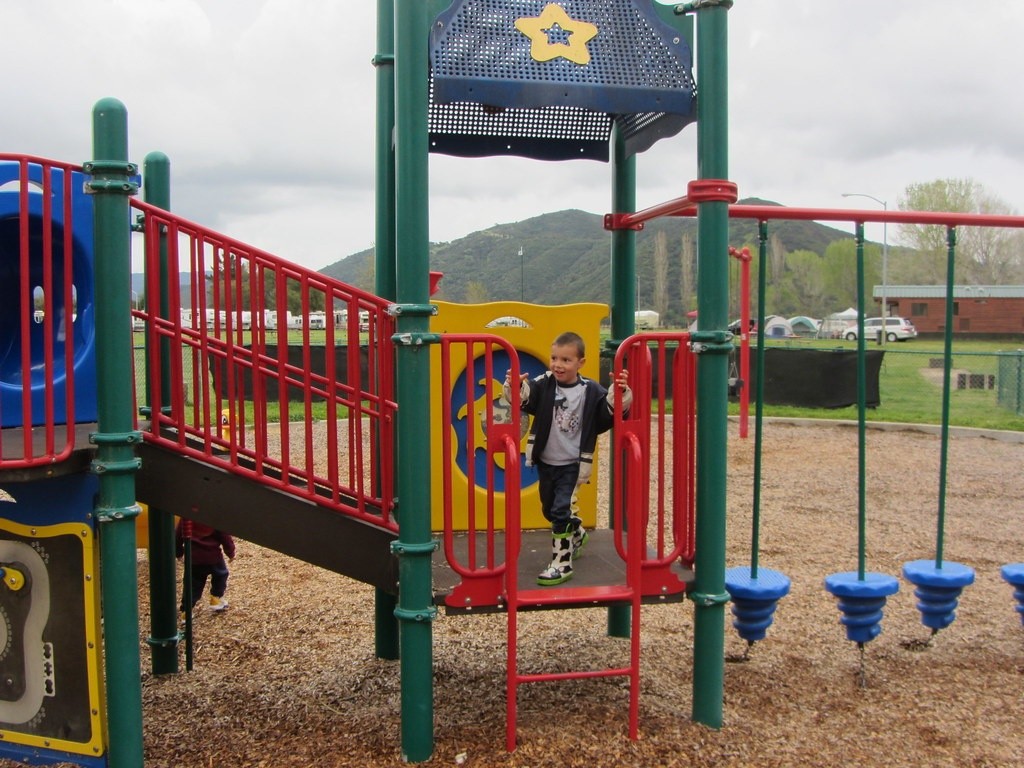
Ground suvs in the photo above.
[840,316,919,342]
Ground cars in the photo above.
[729,319,754,335]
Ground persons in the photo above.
[175,516,236,626]
[502,331,632,588]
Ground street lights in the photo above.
[842,192,887,345]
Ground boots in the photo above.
[570,515,589,560]
[537,523,576,586]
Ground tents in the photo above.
[759,307,869,340]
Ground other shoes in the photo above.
[180,612,194,624]
[210,595,229,611]
